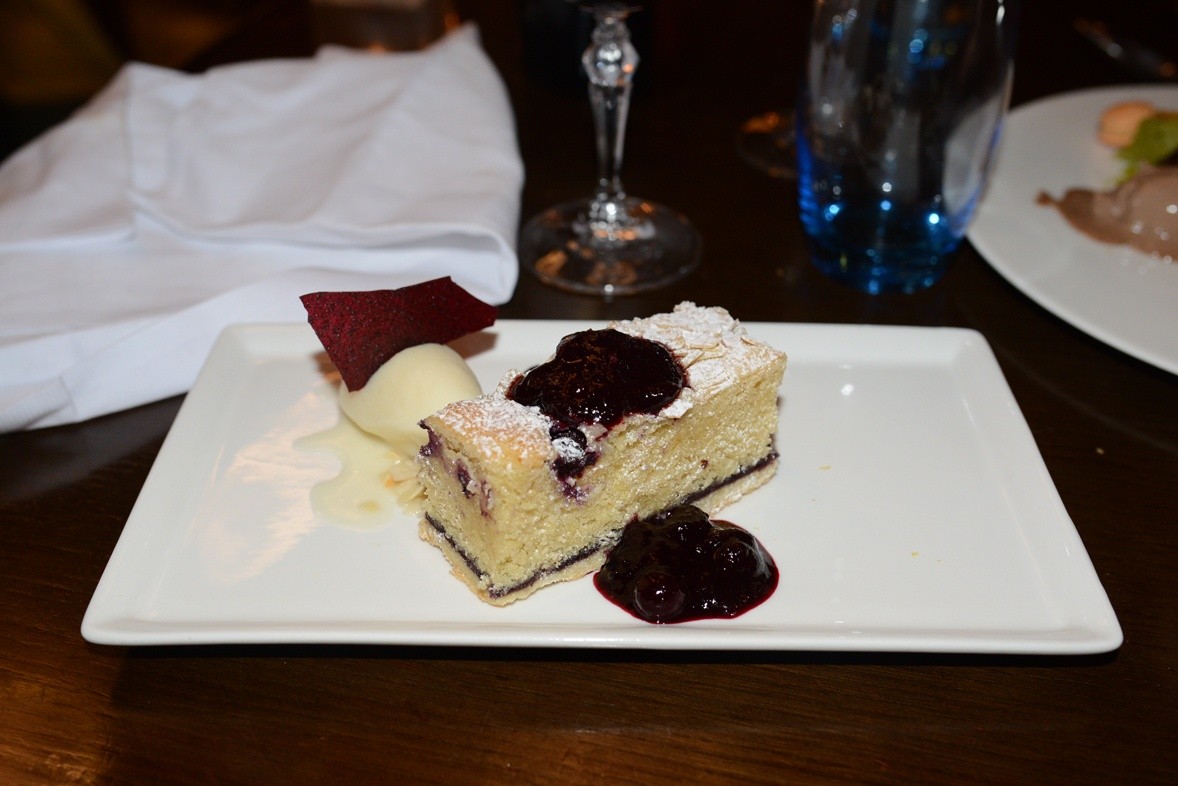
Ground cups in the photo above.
[799,1,1016,305]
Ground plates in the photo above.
[940,84,1178,378]
[80,316,1123,655]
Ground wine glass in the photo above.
[516,1,703,297]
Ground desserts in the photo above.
[296,277,500,446]
[417,302,788,605]
